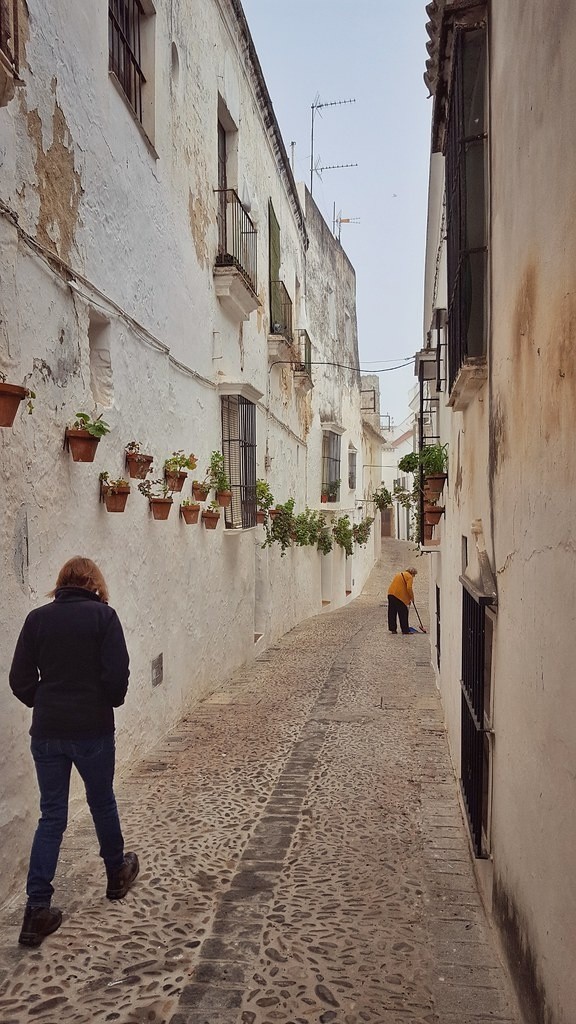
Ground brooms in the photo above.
[400,572,426,634]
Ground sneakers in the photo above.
[18,907,62,948]
[106,852,140,900]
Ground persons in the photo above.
[387,566,417,634]
[8,554,140,949]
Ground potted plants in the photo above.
[0,370,37,428]
[247,440,451,560]
[99,471,130,513]
[163,449,197,492]
[202,501,220,531]
[192,451,227,501]
[125,441,154,479]
[215,476,233,505]
[179,497,200,525]
[139,473,179,520]
[65,411,111,463]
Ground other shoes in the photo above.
[392,631,397,634]
[402,631,410,634]
